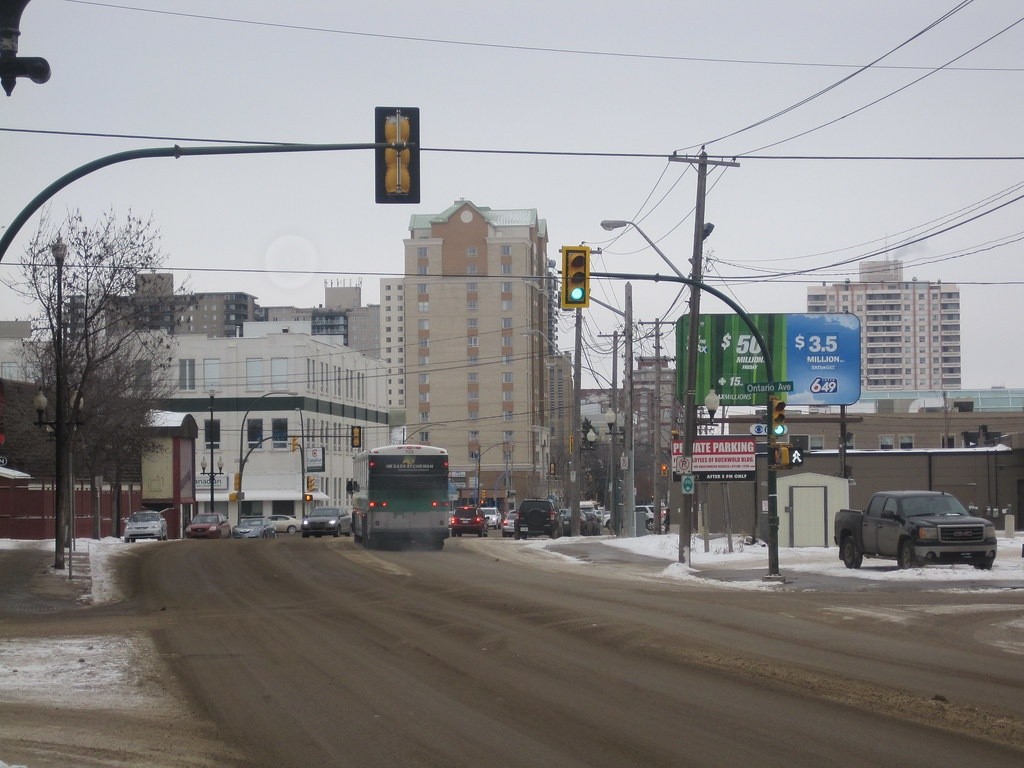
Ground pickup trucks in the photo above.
[834,490,998,572]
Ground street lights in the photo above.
[50,237,67,568]
[478,441,512,507]
[238,390,298,525]
[600,219,720,565]
[200,384,224,513]
[32,386,86,548]
[403,423,447,444]
[520,329,597,539]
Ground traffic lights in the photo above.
[662,465,668,477]
[772,398,786,437]
[291,437,297,452]
[570,436,573,455]
[305,495,313,502]
[561,245,590,309]
[307,476,316,493]
[618,426,626,447]
[788,447,805,467]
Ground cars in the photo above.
[300,506,352,539]
[448,511,457,528]
[231,518,279,539]
[264,515,302,535]
[123,511,168,544]
[501,500,666,539]
[186,513,231,539]
[480,507,502,530]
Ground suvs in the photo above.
[451,507,490,537]
[513,498,567,540]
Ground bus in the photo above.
[352,444,450,551]
